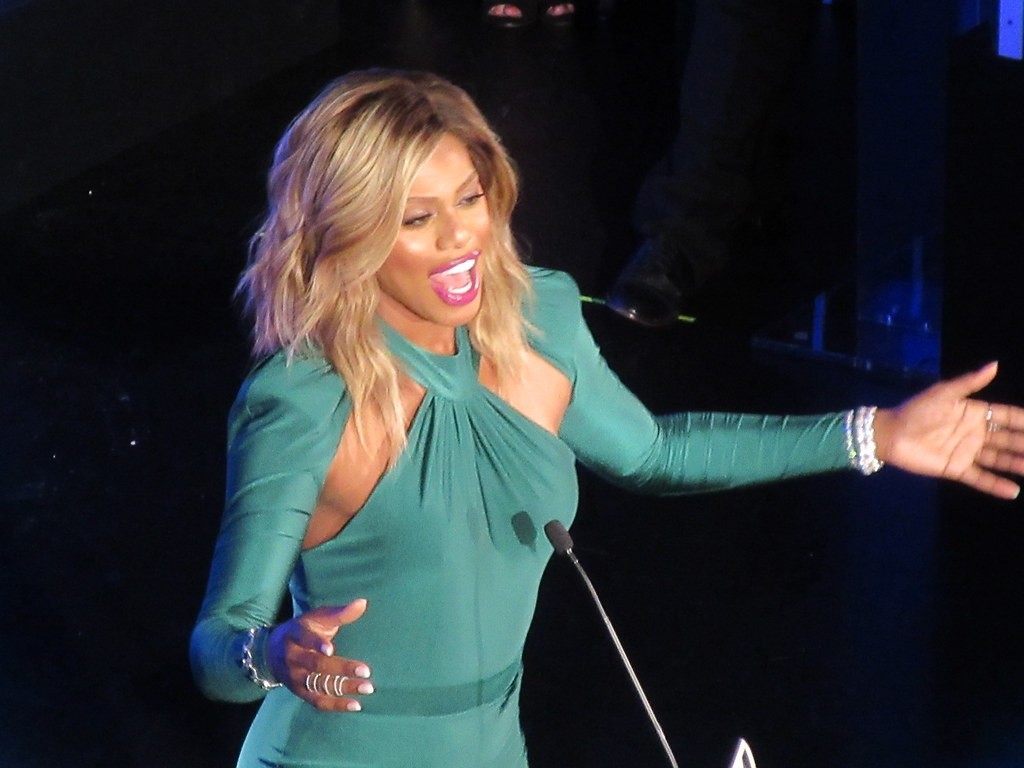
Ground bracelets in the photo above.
[854,405,884,475]
[238,624,285,689]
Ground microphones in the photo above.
[545,520,678,768]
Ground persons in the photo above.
[605,0,810,329]
[180,67,1023,768]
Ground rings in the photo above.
[984,401,995,432]
[305,670,349,697]
